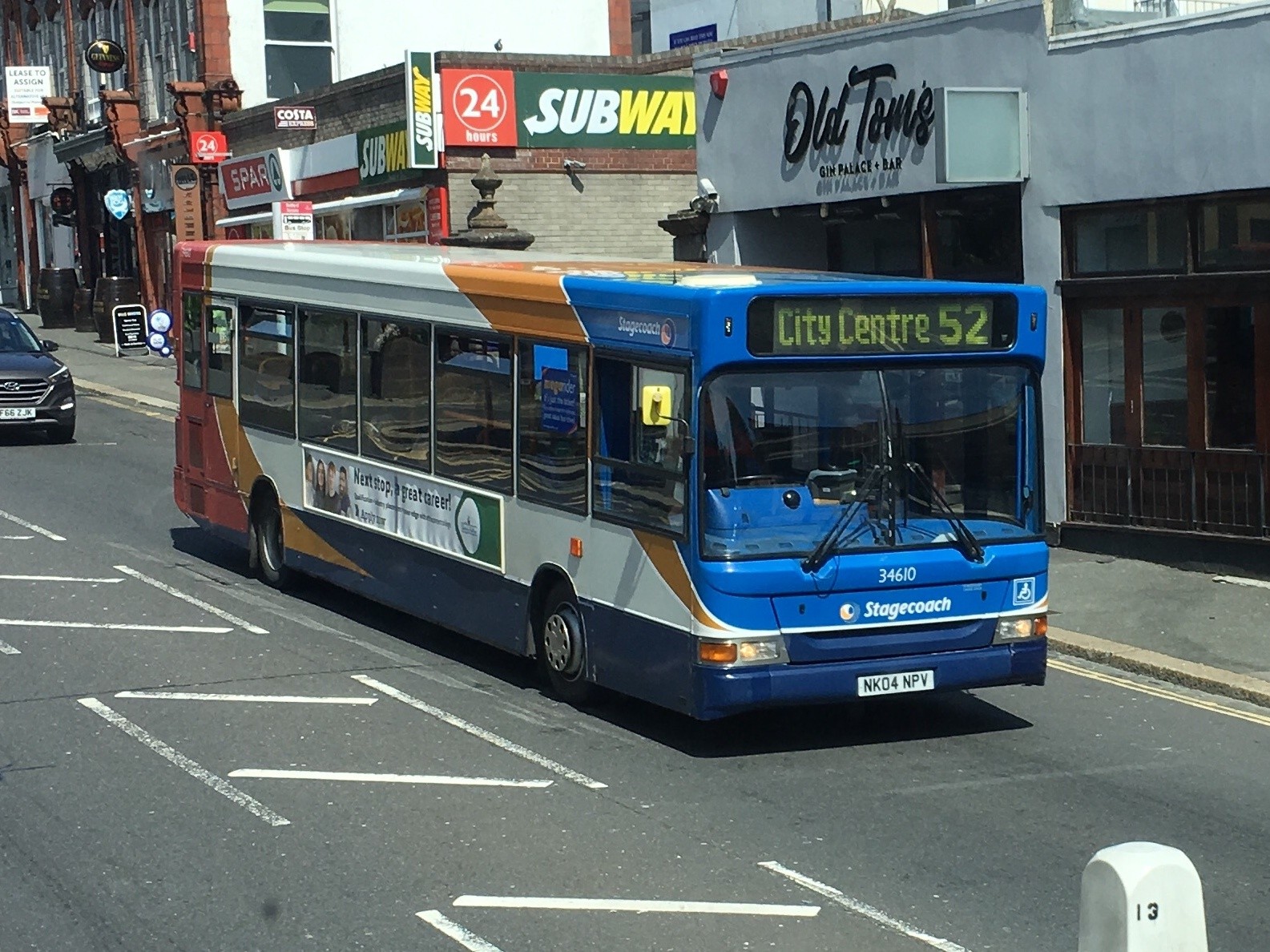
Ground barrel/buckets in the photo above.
[73,287,98,332]
[36,267,81,328]
[93,276,140,342]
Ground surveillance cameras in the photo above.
[699,179,718,200]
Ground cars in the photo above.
[0,307,77,443]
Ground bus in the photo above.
[167,233,1051,724]
